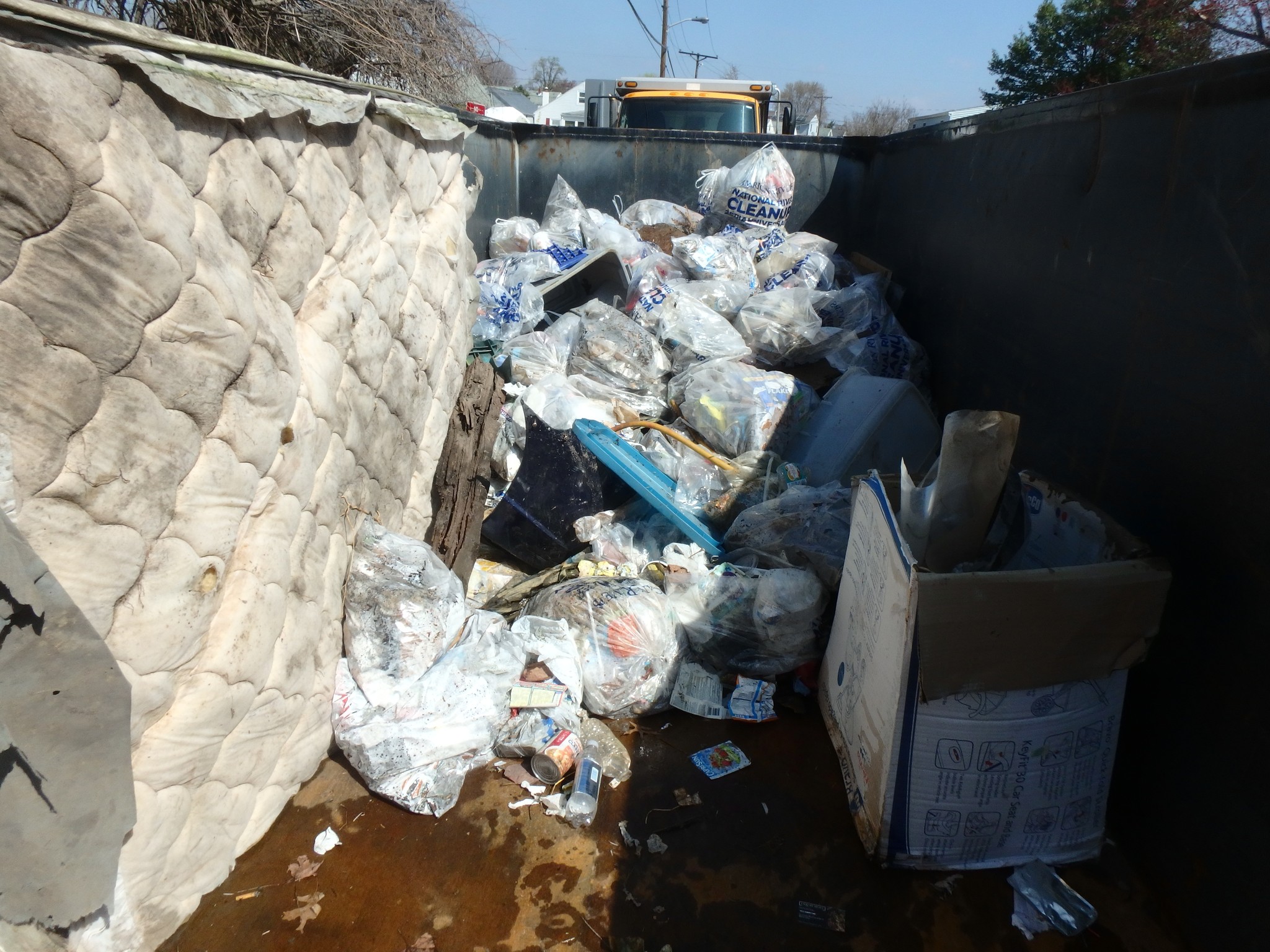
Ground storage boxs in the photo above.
[820,462,1172,870]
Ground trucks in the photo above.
[578,77,796,135]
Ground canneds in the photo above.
[531,729,583,785]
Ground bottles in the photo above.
[564,740,603,828]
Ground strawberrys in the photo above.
[709,748,732,767]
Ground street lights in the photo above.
[660,16,710,77]
